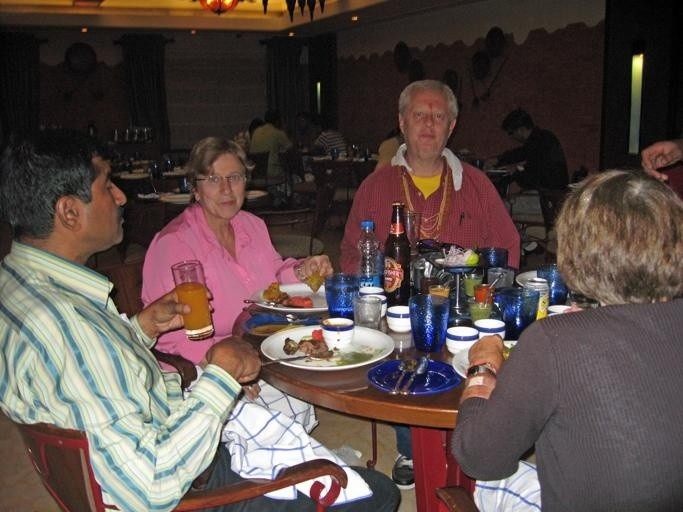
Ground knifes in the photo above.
[399,356,430,395]
[258,351,306,371]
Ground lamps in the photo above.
[190,0,250,16]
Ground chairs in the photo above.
[435,487,481,512]
[93,261,200,397]
[16,420,349,512]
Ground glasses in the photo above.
[194,173,244,185]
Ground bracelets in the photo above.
[297,260,305,278]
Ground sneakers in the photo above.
[392,454,415,490]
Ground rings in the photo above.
[247,384,255,390]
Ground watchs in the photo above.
[467,363,498,379]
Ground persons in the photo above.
[484,108,570,268]
[139,133,335,433]
[449,170,682,510]
[229,107,401,218]
[338,77,522,490]
[640,135,682,182]
[0,119,403,512]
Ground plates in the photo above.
[250,281,328,314]
[242,311,318,340]
[514,267,536,286]
[260,324,394,372]
[366,359,462,397]
[453,340,520,380]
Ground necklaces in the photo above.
[402,175,448,243]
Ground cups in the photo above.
[487,268,515,290]
[468,303,493,321]
[324,273,361,316]
[321,317,355,346]
[480,247,508,268]
[170,258,216,343]
[447,325,478,354]
[501,286,541,336]
[386,306,413,334]
[474,318,507,341]
[538,263,569,305]
[364,293,387,319]
[473,284,496,303]
[353,294,382,332]
[464,275,482,297]
[408,293,449,353]
[429,284,449,300]
[359,286,385,294]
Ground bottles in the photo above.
[383,201,412,307]
[356,220,382,286]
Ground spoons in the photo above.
[387,357,417,396]
